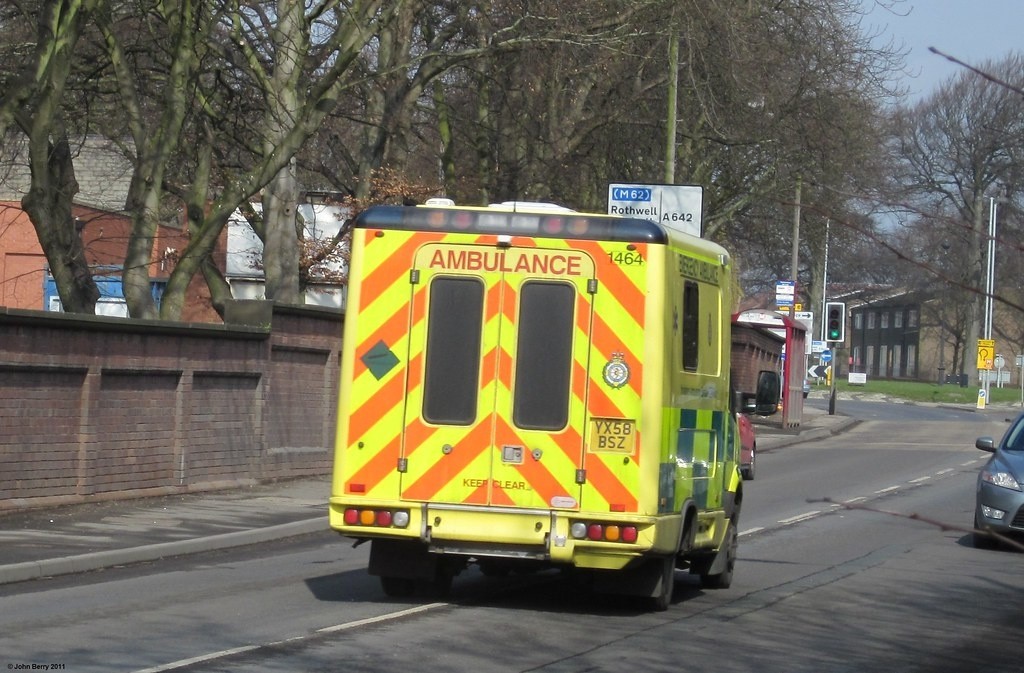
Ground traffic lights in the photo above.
[825,302,845,343]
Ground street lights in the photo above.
[816,213,831,386]
[976,195,1009,409]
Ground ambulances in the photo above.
[327,197,781,614]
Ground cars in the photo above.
[975,409,1024,545]
